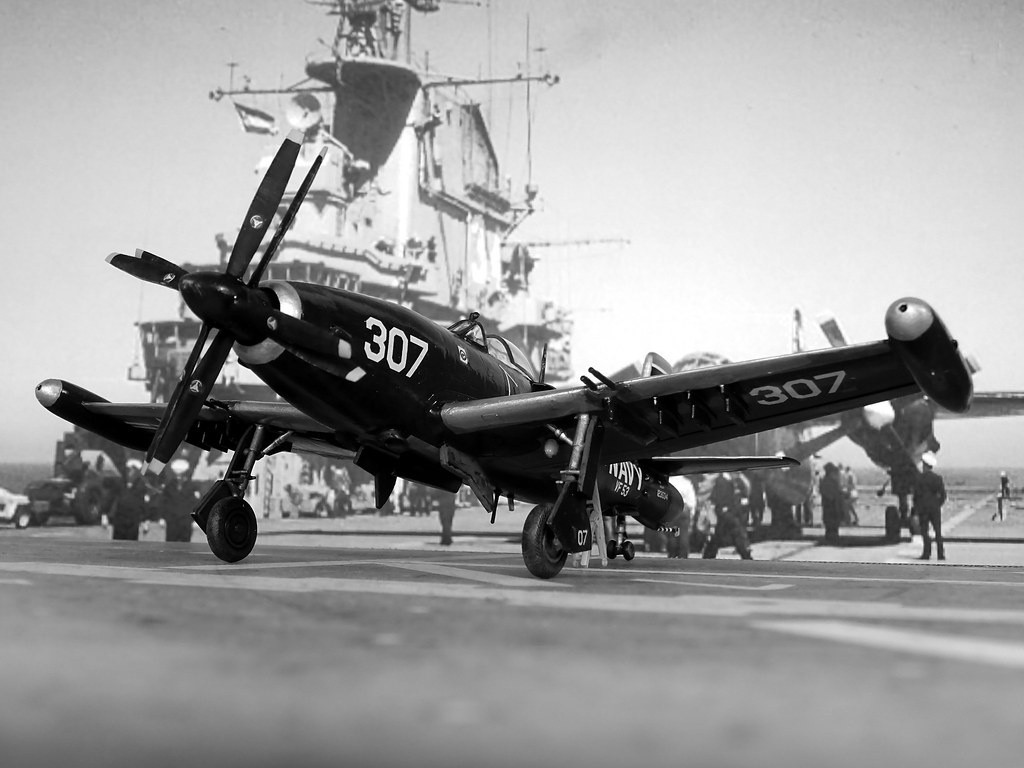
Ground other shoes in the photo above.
[918,555,929,559]
[938,557,945,560]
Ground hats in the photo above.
[921,453,937,469]
[823,462,838,477]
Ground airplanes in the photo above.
[32,124,983,579]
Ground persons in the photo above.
[409,481,455,545]
[820,463,859,540]
[913,451,946,560]
[331,460,355,516]
[643,470,764,560]
